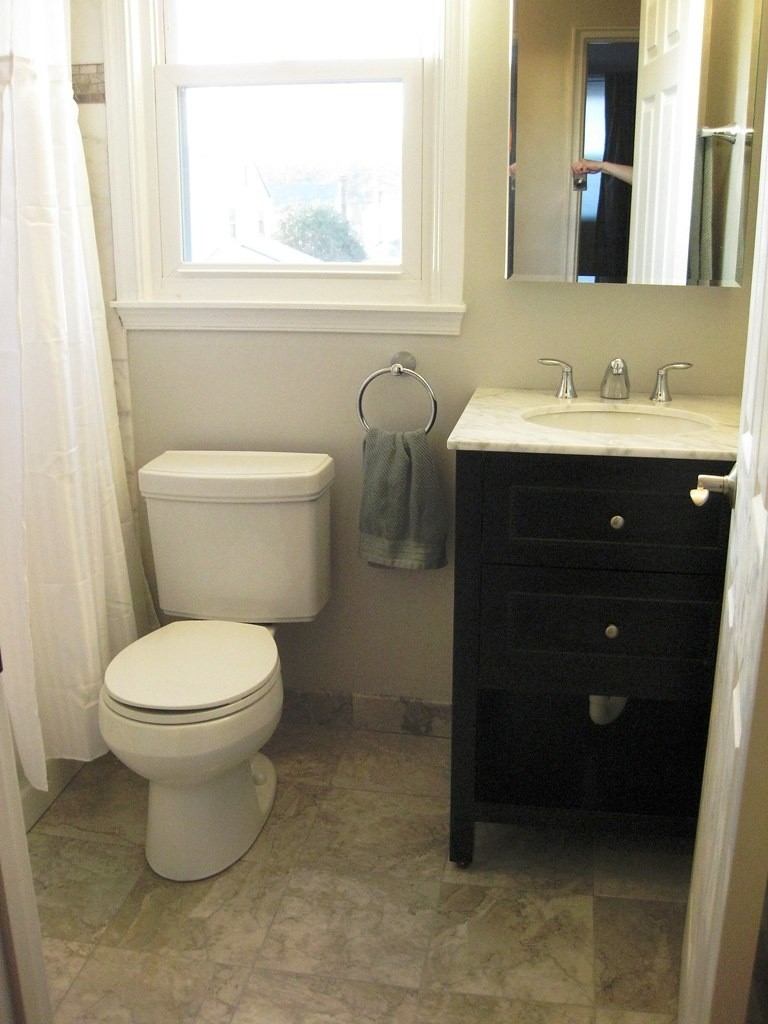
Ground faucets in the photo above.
[600,358,630,400]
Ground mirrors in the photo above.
[504,0,762,288]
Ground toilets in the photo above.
[98,452,336,883]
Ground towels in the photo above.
[685,124,712,287]
[357,424,450,572]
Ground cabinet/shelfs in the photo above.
[451,449,738,866]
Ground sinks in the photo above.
[520,404,717,435]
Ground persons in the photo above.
[572,158,633,187]
